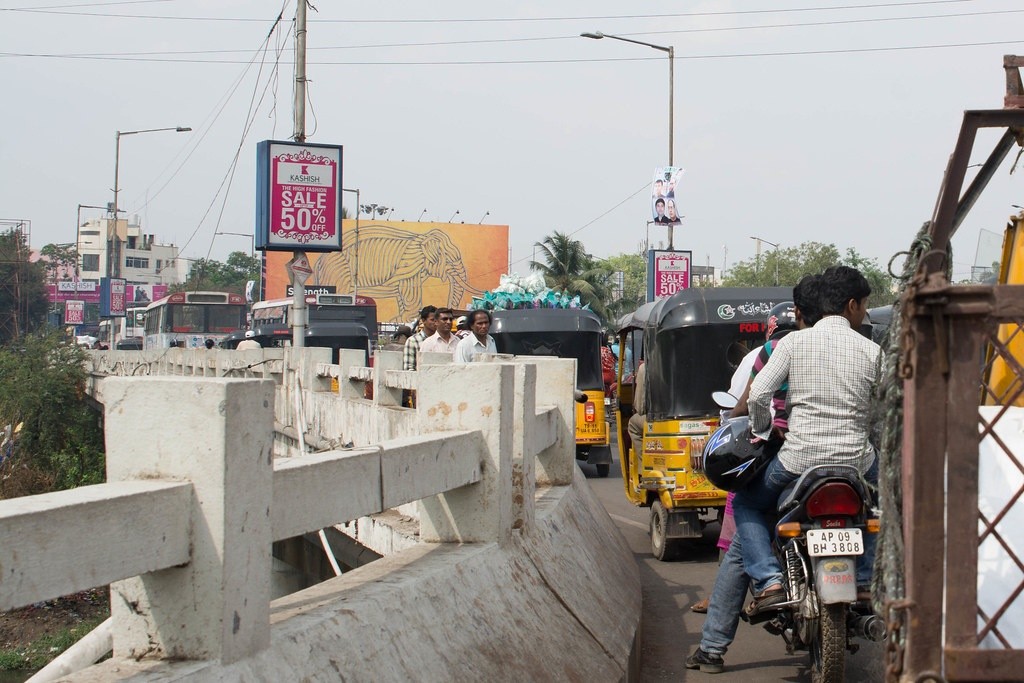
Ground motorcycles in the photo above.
[217,320,372,399]
[707,387,892,683]
[451,277,910,560]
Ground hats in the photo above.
[456,315,469,327]
[245,331,255,338]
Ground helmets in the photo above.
[703,416,783,492]
[206,339,214,349]
[765,302,801,340]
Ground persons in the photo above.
[456,309,497,362]
[135,286,150,301]
[612,339,632,375]
[629,363,646,483]
[236,331,261,350]
[731,267,887,615]
[420,307,461,353]
[653,180,679,222]
[601,333,618,383]
[455,315,471,340]
[685,273,823,671]
[404,305,437,371]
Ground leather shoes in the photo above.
[686,648,724,672]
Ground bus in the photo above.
[140,291,246,352]
[96,304,148,352]
[244,291,380,401]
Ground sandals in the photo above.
[852,588,872,612]
[690,600,709,612]
[746,587,786,617]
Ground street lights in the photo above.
[579,29,676,250]
[108,126,193,351]
